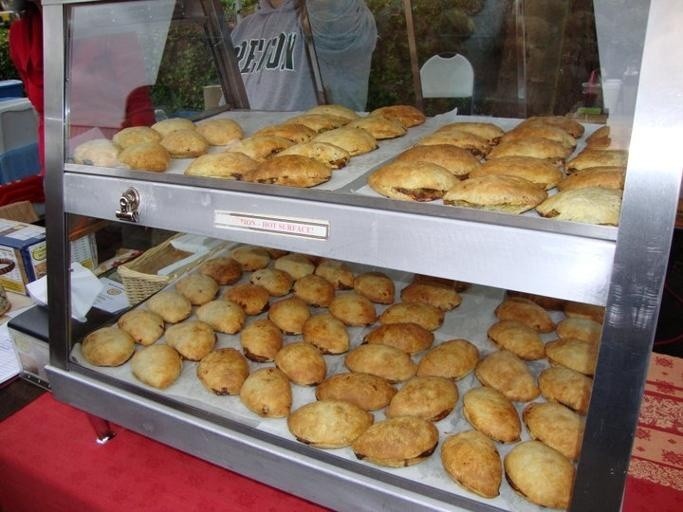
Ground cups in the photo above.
[581,81,602,109]
[202,84,223,113]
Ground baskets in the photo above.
[116,233,233,308]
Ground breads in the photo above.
[80,244,605,507]
[73,105,629,224]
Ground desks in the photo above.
[0,350,683,512]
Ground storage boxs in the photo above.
[0,201,99,298]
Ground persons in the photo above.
[0,0,155,219]
[207,0,377,122]
